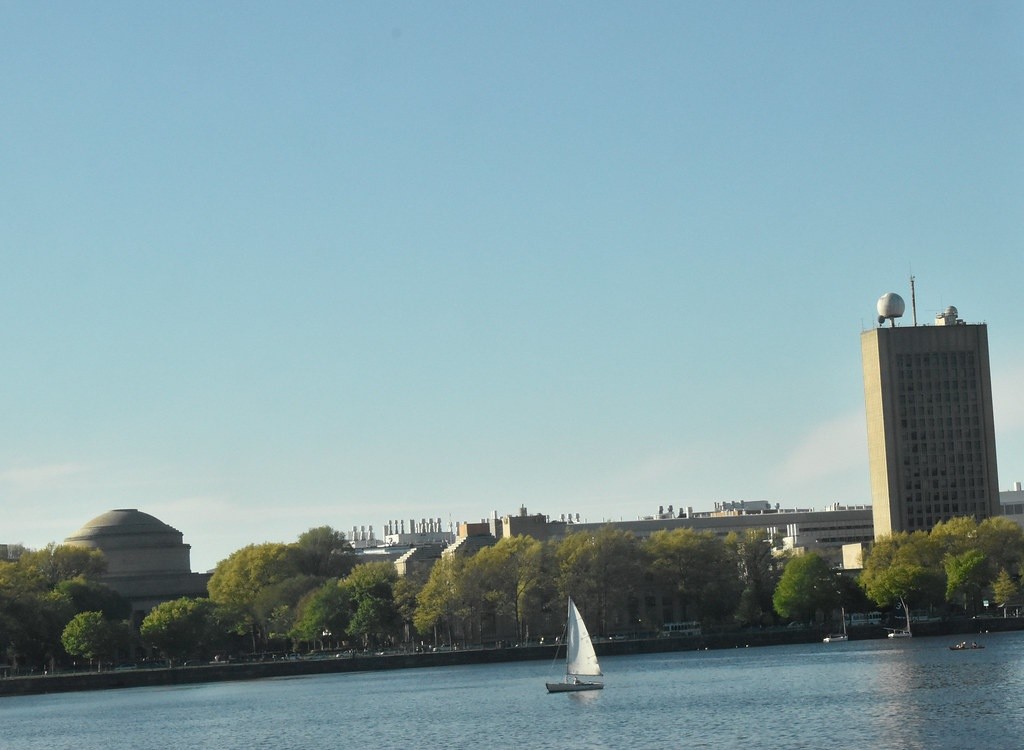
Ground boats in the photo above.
[948,639,985,651]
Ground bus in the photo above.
[657,621,701,637]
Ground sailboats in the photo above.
[882,596,913,638]
[822,607,848,644]
[544,596,605,693]
